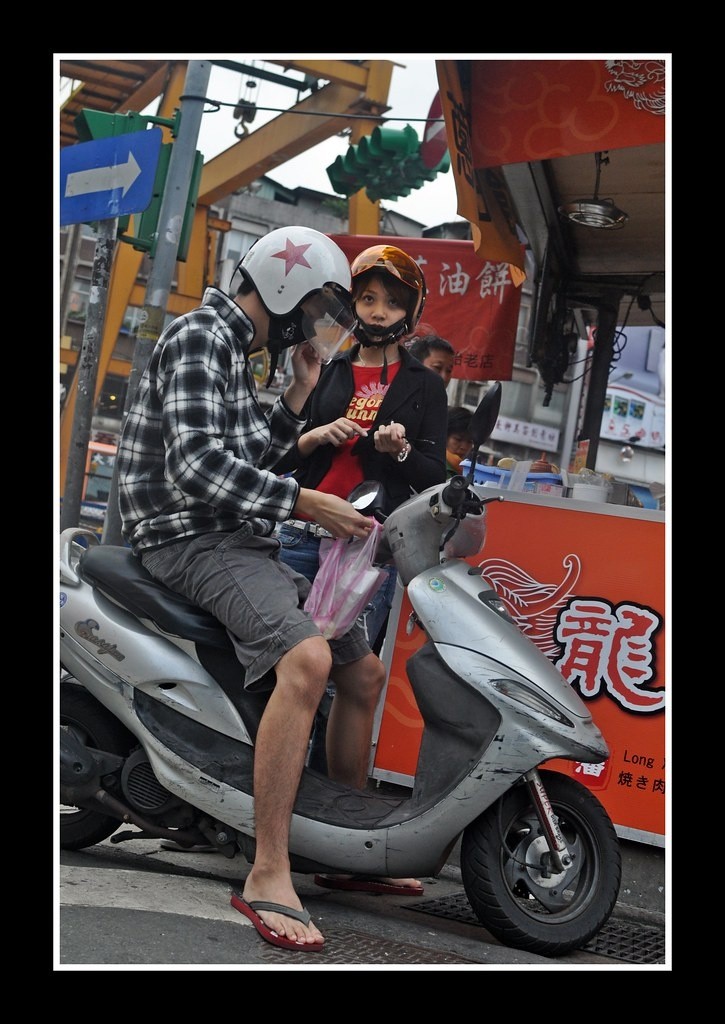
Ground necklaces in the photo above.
[356,352,400,367]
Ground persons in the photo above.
[409,337,454,391]
[118,225,426,953]
[446,406,475,476]
[277,245,449,777]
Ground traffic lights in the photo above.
[71,106,148,143]
[326,123,419,199]
[364,141,451,201]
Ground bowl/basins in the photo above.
[572,483,609,503]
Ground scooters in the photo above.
[59,375,624,959]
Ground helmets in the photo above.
[351,245,426,350]
[228,225,352,320]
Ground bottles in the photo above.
[530,452,552,473]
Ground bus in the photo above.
[69,438,117,544]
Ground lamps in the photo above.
[558,150,629,230]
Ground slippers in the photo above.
[313,872,424,895]
[229,893,326,952]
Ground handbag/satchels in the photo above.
[304,516,389,641]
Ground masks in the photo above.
[275,307,317,353]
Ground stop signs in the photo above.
[422,90,449,170]
